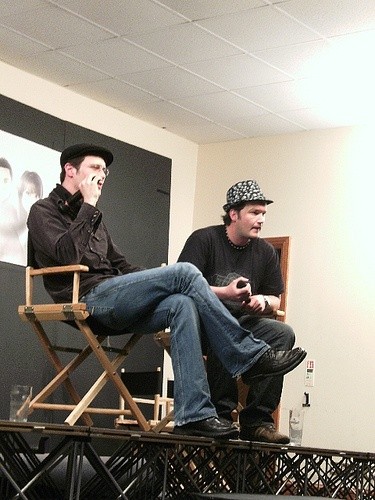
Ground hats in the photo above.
[60,143,113,167]
[222,180,273,212]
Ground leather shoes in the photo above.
[241,347,306,385]
[173,415,240,439]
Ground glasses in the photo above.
[75,163,110,176]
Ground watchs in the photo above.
[263,296,270,310]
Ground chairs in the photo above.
[16,263,285,433]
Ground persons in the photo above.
[27,143,308,439]
[176,180,295,445]
[0,157,43,268]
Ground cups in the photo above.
[288,410,304,447]
[8,384,32,422]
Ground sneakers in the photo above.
[239,424,290,443]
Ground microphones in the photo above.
[58,177,99,211]
[238,281,250,304]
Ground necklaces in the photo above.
[225,225,251,249]
